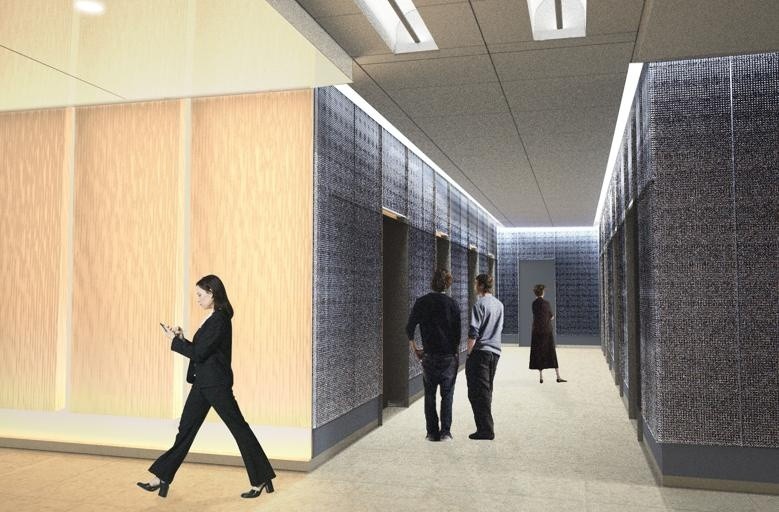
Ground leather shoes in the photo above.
[469,432,495,440]
[557,379,566,382]
[540,379,543,383]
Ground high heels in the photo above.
[137,477,169,497]
[242,478,274,498]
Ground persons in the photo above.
[466,273,507,440]
[136,273,277,501]
[404,268,462,442]
[528,284,568,383]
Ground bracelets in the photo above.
[466,354,470,357]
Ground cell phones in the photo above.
[160,323,167,332]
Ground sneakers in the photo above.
[440,432,452,440]
